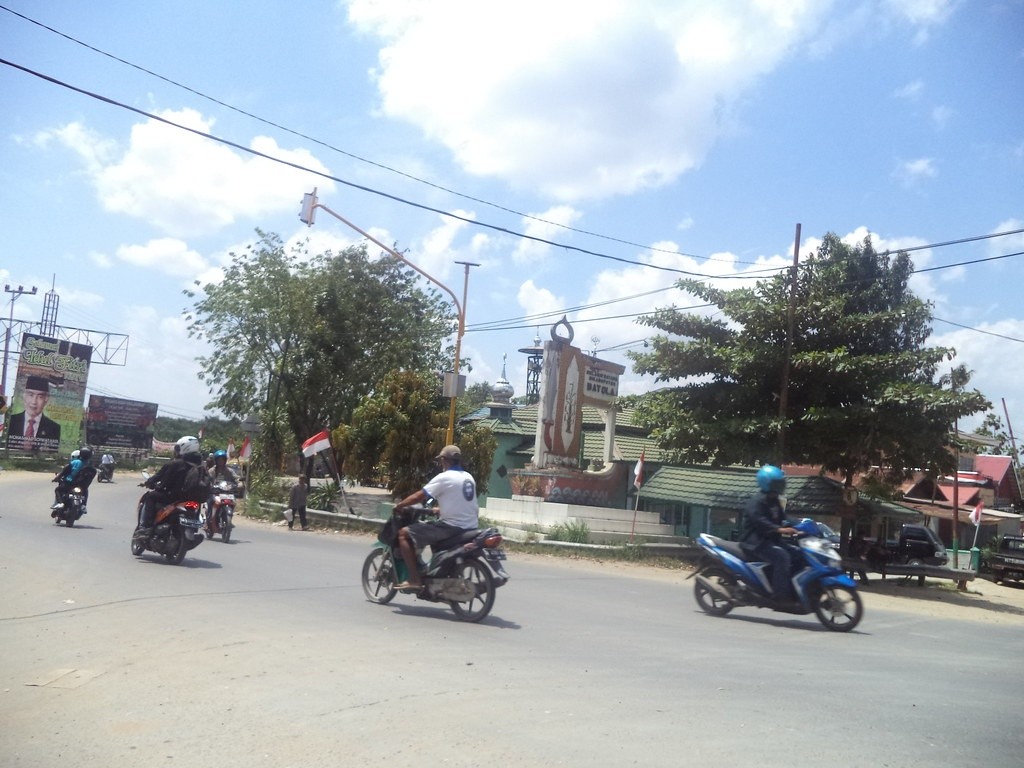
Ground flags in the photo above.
[198,424,204,438]
[301,429,331,458]
[240,436,252,459]
[968,501,985,527]
[227,437,235,463]
[634,444,645,491]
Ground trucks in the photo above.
[989,534,1024,585]
[847,527,948,569]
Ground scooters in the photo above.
[685,520,864,632]
[130,471,205,565]
[362,497,508,621]
[98,460,119,482]
[53,470,87,527]
[204,474,248,543]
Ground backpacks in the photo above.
[184,460,211,504]
[71,461,97,488]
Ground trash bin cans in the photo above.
[969,546,988,574]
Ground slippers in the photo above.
[393,581,426,589]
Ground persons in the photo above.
[6,375,61,452]
[134,436,202,534]
[392,445,478,588]
[739,465,802,608]
[288,474,310,531]
[205,449,244,529]
[102,448,115,475]
[50,447,92,514]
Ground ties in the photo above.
[23,418,36,450]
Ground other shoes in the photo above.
[302,526,309,530]
[135,527,152,535]
[289,528,294,531]
[49,503,57,508]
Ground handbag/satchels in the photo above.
[283,508,293,521]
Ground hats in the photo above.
[435,445,461,459]
[26,376,50,391]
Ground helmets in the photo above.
[213,449,227,460]
[71,450,80,460]
[174,436,199,457]
[757,465,786,496]
[79,446,94,459]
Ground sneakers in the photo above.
[81,505,87,512]
[52,503,64,509]
